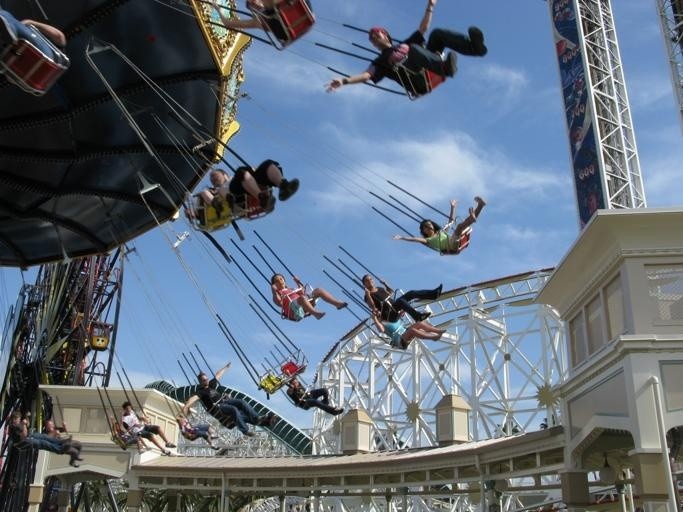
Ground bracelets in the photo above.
[425,8,433,12]
[342,78,347,84]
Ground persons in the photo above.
[44,419,83,468]
[175,414,220,451]
[322,0,488,93]
[121,402,175,455]
[1,8,66,64]
[209,159,298,213]
[362,273,442,323]
[370,309,446,348]
[210,0,312,40]
[184,187,222,221]
[287,378,343,417]
[390,196,486,253]
[111,416,152,455]
[182,363,276,437]
[10,412,71,455]
[270,273,348,322]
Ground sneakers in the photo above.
[436,284,442,299]
[278,178,300,202]
[467,26,488,57]
[334,408,344,416]
[161,449,173,456]
[165,442,176,448]
[264,195,276,214]
[255,413,268,424]
[415,312,432,323]
[245,431,256,437]
[444,52,458,78]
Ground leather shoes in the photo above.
[209,434,219,438]
[210,445,220,450]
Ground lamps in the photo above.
[492,463,509,493]
[598,450,618,484]
